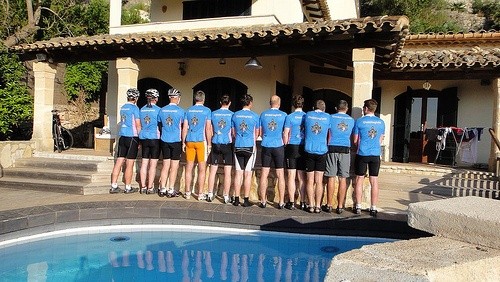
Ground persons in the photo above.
[257,95,289,209]
[282,95,308,212]
[305,99,331,213]
[107,244,330,282]
[230,94,260,207]
[353,99,385,218]
[157,88,183,198]
[108,88,141,194]
[206,95,233,203]
[181,90,212,201]
[138,89,162,195]
[321,101,356,214]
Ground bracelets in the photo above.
[208,145,212,149]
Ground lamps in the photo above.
[177,59,187,76]
[243,55,264,71]
[218,57,226,65]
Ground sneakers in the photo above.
[110,187,382,217]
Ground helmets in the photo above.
[168,89,181,98]
[144,88,159,99]
[127,89,140,97]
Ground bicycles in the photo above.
[50,109,73,153]
